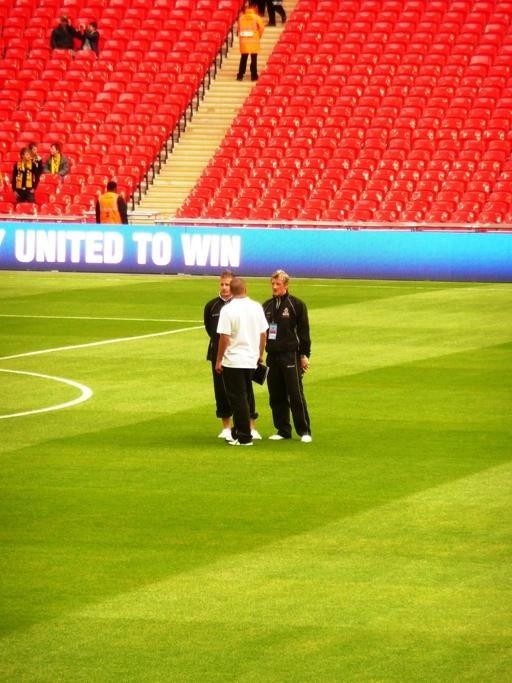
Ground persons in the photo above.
[204,272,262,439]
[236,0,286,81]
[262,269,312,442]
[215,278,270,445]
[49,16,78,51]
[77,23,100,55]
[12,144,70,202]
[95,181,128,224]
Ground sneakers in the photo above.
[268,434,284,440]
[216,428,263,446]
[300,434,312,442]
